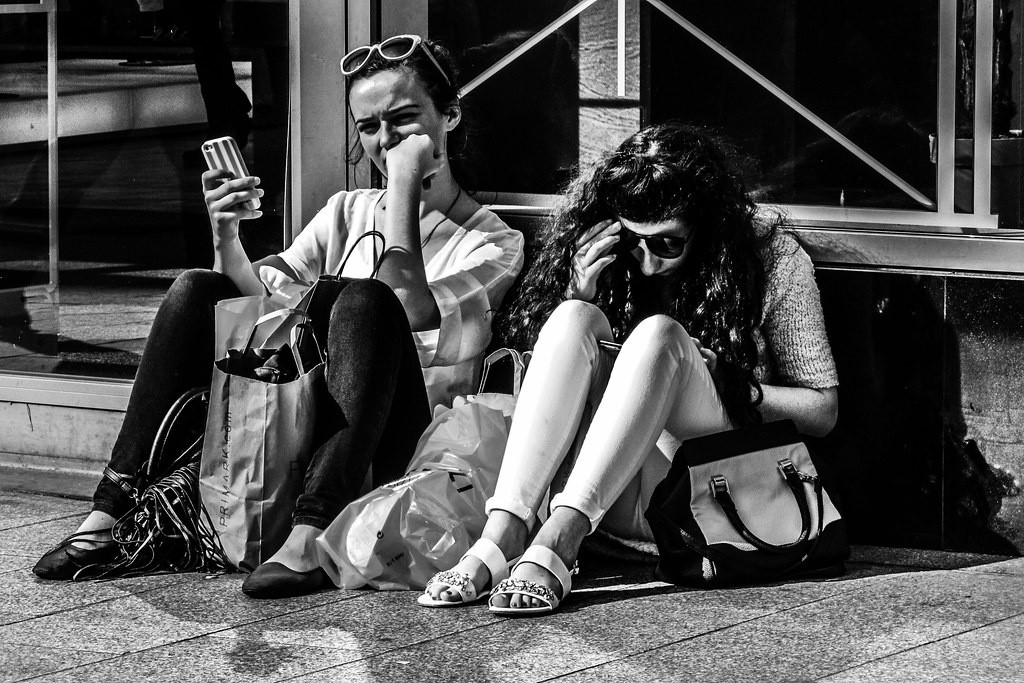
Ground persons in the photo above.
[0,9,154,356]
[174,0,290,197]
[31,29,525,595]
[454,32,581,192]
[758,116,933,210]
[419,124,841,611]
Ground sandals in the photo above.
[488,545,571,613]
[417,538,523,607]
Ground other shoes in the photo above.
[32,542,121,580]
[242,563,339,596]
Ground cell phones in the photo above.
[598,340,622,356]
[201,135,261,210]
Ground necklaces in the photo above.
[420,187,462,246]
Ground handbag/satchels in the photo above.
[109,230,386,570]
[405,402,507,498]
[645,418,852,590]
[313,463,487,593]
[466,345,534,432]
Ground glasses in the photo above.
[619,226,688,259]
[340,34,450,85]
